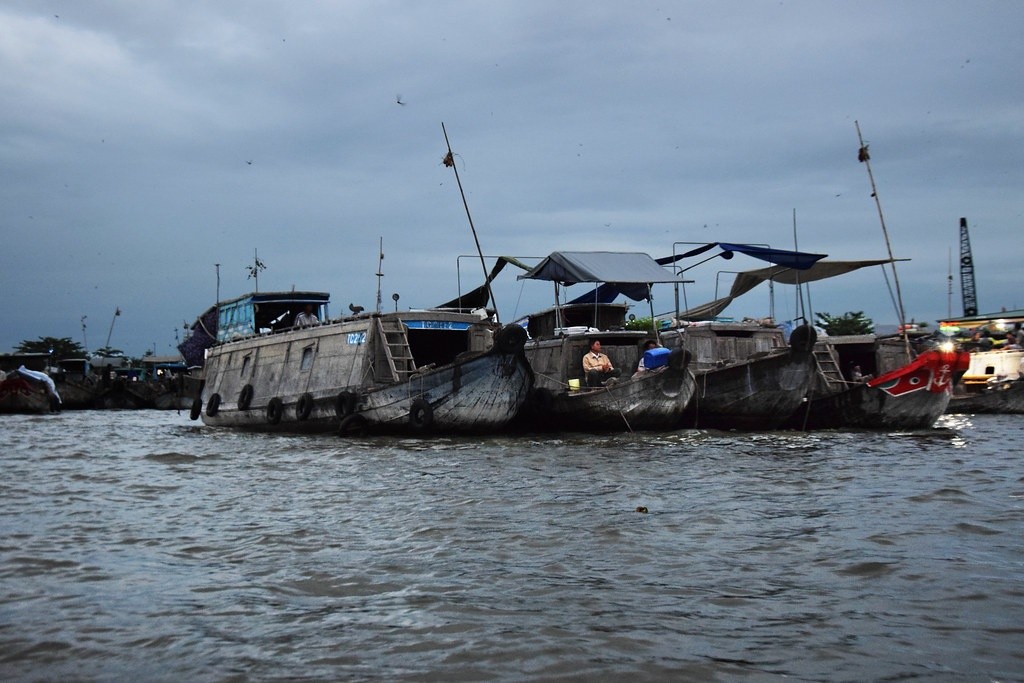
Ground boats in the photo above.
[176,235,535,435]
[653,240,1024,432]
[516,246,697,431]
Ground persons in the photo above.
[583,338,622,387]
[1000,330,1024,351]
[637,338,657,371]
[969,331,980,352]
[295,305,321,324]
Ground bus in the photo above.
[0,353,188,384]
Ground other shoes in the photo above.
[603,377,617,385]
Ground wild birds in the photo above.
[397,95,407,106]
[349,303,364,315]
